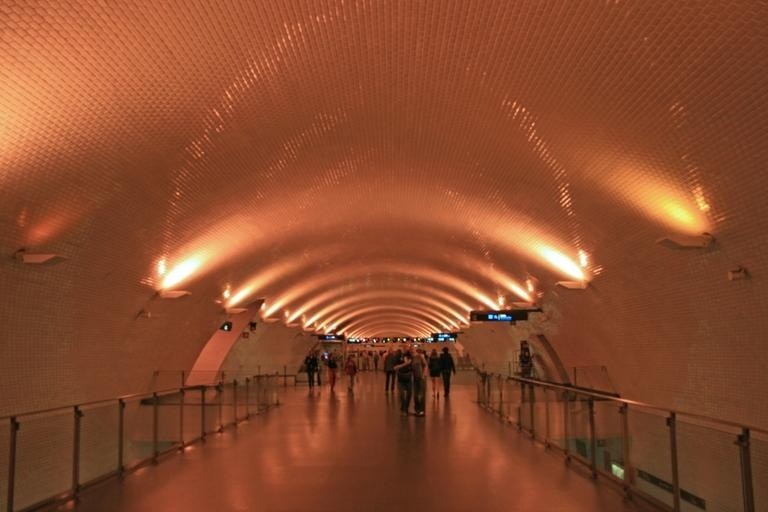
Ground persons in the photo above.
[383,347,455,398]
[374,352,379,370]
[325,352,338,392]
[345,355,360,392]
[304,350,318,389]
[361,350,368,369]
[393,349,413,416]
[519,351,531,376]
[409,349,428,417]
